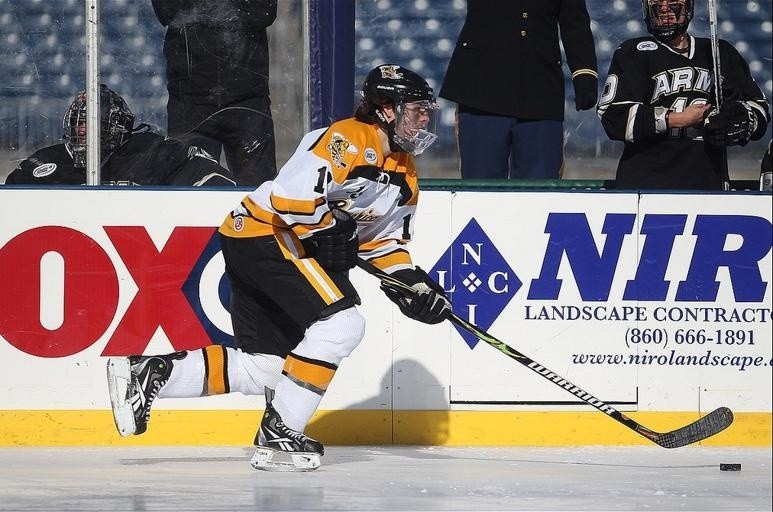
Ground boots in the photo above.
[128,349,187,435]
[254,385,325,455]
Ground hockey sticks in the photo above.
[353,255,733,449]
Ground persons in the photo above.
[150,1,278,188]
[438,0,601,181]
[594,0,772,191]
[127,62,456,458]
[4,82,239,188]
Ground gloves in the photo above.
[703,100,759,146]
[381,266,452,325]
[303,216,359,272]
[573,90,597,110]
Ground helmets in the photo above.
[362,64,436,104]
[64,85,134,119]
